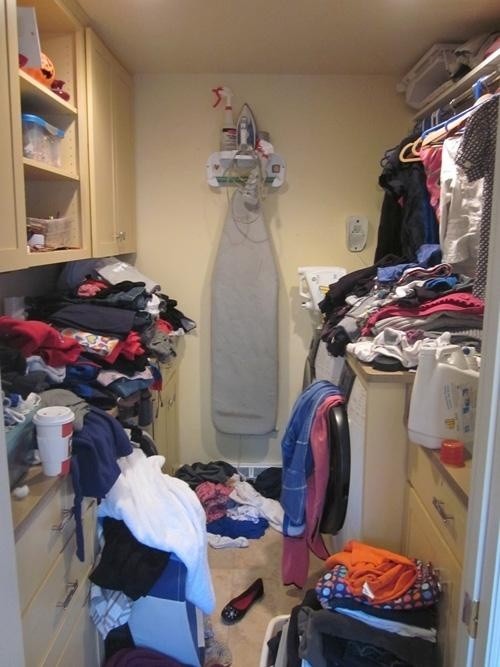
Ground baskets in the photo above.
[26,216,70,250]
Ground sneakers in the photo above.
[220,577,264,626]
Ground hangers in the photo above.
[380,76,500,168]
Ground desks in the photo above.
[315,322,416,556]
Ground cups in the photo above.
[32,405,75,478]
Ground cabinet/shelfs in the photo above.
[403,417,472,667]
